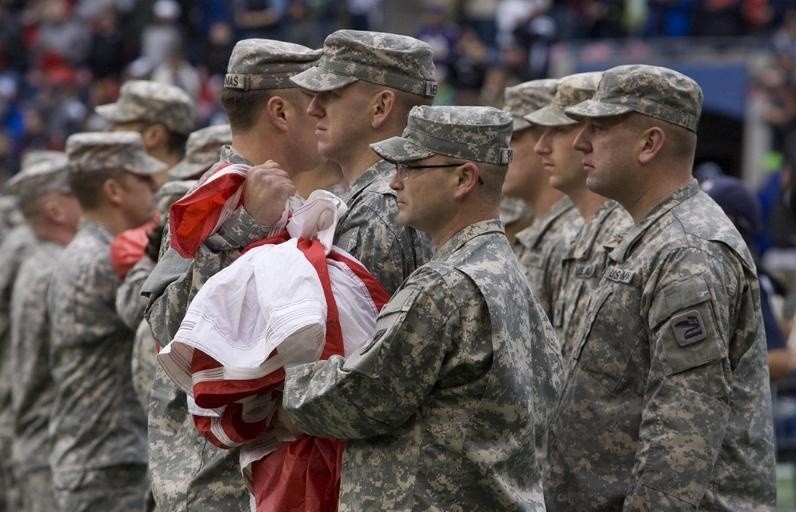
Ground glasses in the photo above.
[395,162,484,185]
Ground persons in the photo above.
[0,0,795,512]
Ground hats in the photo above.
[502,78,558,132]
[94,80,190,134]
[369,105,514,166]
[6,156,69,203]
[64,131,168,175]
[523,71,604,128]
[288,29,439,98]
[168,123,232,179]
[564,63,705,134]
[223,37,324,91]
